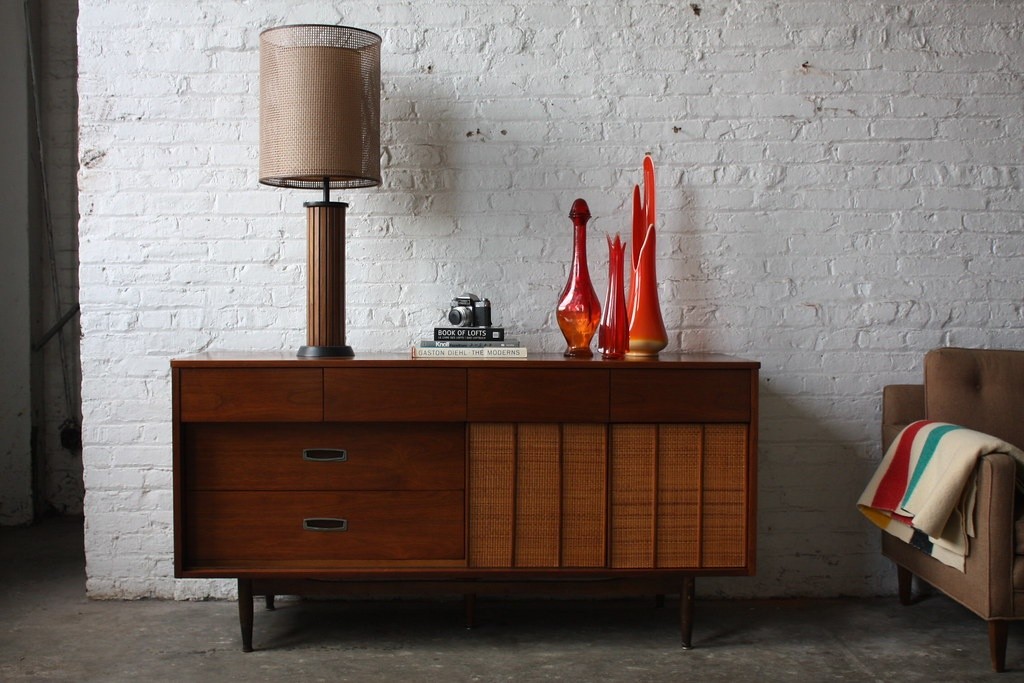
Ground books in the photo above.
[411,327,528,359]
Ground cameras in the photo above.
[448,293,492,328]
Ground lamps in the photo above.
[259,22,385,360]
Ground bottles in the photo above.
[556,199,601,357]
[625,155,668,358]
[599,233,628,359]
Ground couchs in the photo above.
[878,347,1024,674]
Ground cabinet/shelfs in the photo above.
[169,350,762,654]
